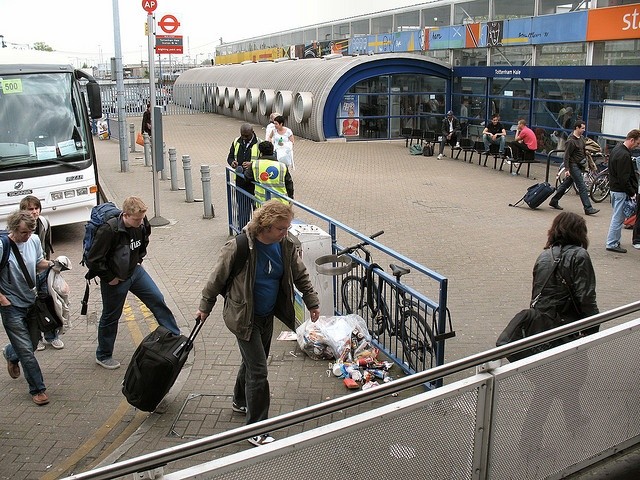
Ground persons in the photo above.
[519,213,600,458]
[606,129,640,253]
[141,104,151,166]
[482,115,506,158]
[245,141,294,208]
[633,156,640,249]
[87,197,180,369]
[0,211,55,405]
[460,99,469,137]
[195,199,320,447]
[20,196,64,351]
[507,120,538,162]
[265,113,281,144]
[342,110,358,135]
[554,107,578,157]
[549,121,600,215]
[437,110,461,160]
[268,116,294,199]
[227,124,265,238]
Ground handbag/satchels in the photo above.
[36,298,62,332]
[411,144,423,155]
[496,308,588,396]
[136,133,144,146]
[579,157,587,171]
[423,145,433,156]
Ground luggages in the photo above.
[508,175,570,208]
[120,315,209,413]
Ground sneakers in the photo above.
[498,151,505,155]
[37,340,44,349]
[3,349,21,380]
[437,154,444,160]
[32,393,50,404]
[585,208,600,215]
[247,435,274,446]
[549,202,563,210]
[483,150,489,154]
[456,140,461,148]
[512,158,519,162]
[230,402,248,413]
[94,357,120,370]
[632,243,640,250]
[52,339,65,350]
[605,245,627,253]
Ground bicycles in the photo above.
[339,230,438,384]
[555,154,640,202]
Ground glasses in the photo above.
[270,224,292,232]
[12,228,36,236]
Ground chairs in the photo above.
[478,125,486,137]
[490,141,501,169]
[421,131,434,147]
[410,128,422,147]
[518,151,540,177]
[448,132,462,159]
[499,147,514,173]
[399,126,412,147]
[460,138,475,163]
[475,141,485,165]
[431,133,442,146]
[466,124,479,139]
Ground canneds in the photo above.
[356,356,373,367]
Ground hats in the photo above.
[446,110,454,115]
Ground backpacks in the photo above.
[80,202,145,316]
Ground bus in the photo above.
[1,50,103,235]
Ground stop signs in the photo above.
[158,15,181,34]
